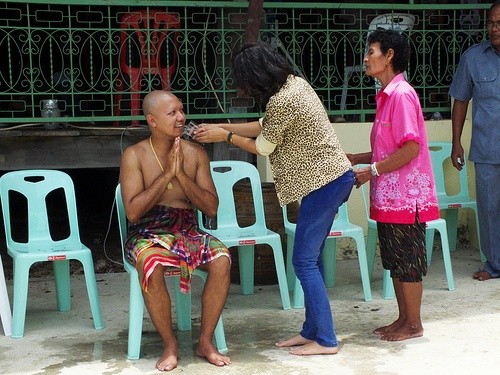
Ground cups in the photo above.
[41,99,60,130]
[183,121,200,139]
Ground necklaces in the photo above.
[150,135,173,190]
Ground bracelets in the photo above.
[370,162,380,177]
[227,131,235,145]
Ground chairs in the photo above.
[0,253,13,336]
[112,9,181,128]
[0,169,106,338]
[114,183,229,362]
[282,200,372,310]
[428,142,487,264]
[358,163,455,301]
[340,13,415,118]
[196,159,292,310]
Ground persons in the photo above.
[342,27,440,341]
[448,3,500,281]
[191,43,354,356]
[121,90,232,372]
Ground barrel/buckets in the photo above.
[229,181,299,284]
